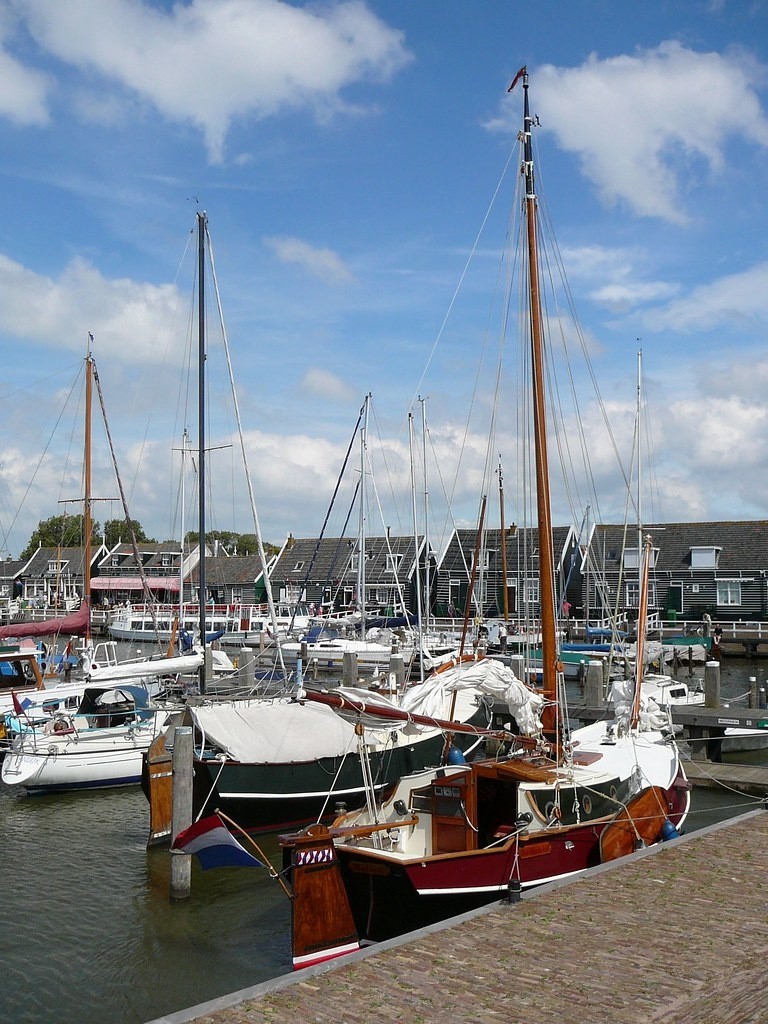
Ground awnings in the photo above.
[90,577,181,593]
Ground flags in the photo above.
[171,814,270,872]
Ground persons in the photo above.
[308,603,323,618]
[209,597,215,604]
[235,599,239,604]
[448,600,456,618]
[713,624,723,644]
[498,623,509,655]
[103,596,130,610]
[703,613,711,626]
[561,599,571,619]
[17,590,78,609]
[476,613,483,625]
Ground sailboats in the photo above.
[0,203,719,833]
[269,61,696,918]
